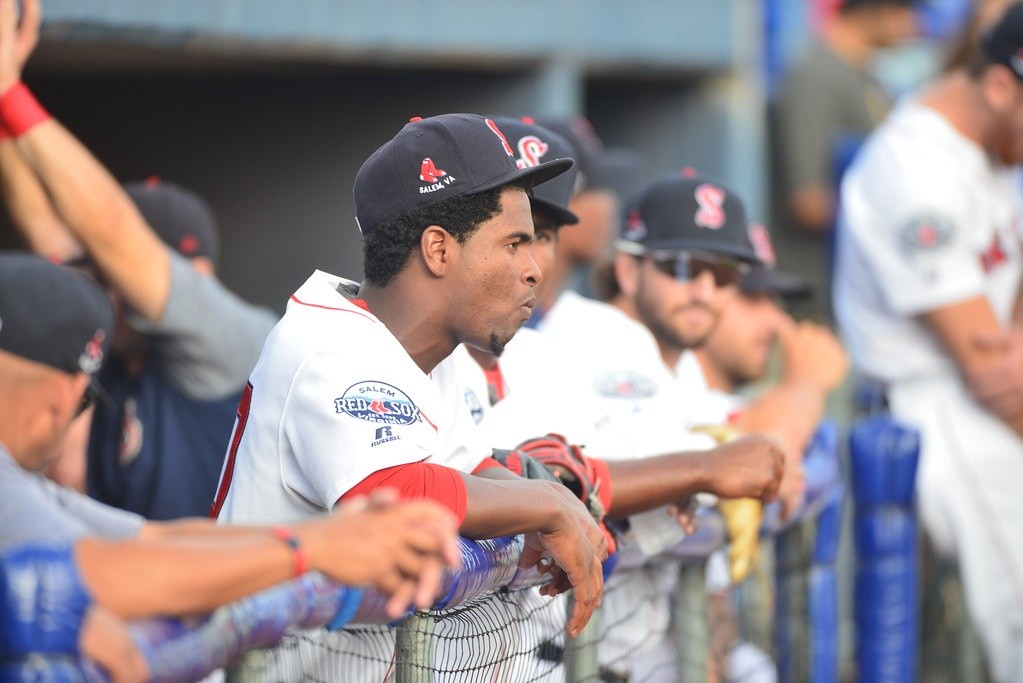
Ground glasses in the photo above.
[622,238,740,290]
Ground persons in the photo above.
[0,0,1023,683]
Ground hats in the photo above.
[63,184,221,281]
[737,223,825,302]
[355,111,573,229]
[0,253,118,414]
[980,1,1023,80]
[494,117,582,235]
[616,178,762,263]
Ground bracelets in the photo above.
[0,81,50,137]
[0,125,10,141]
[269,526,306,579]
